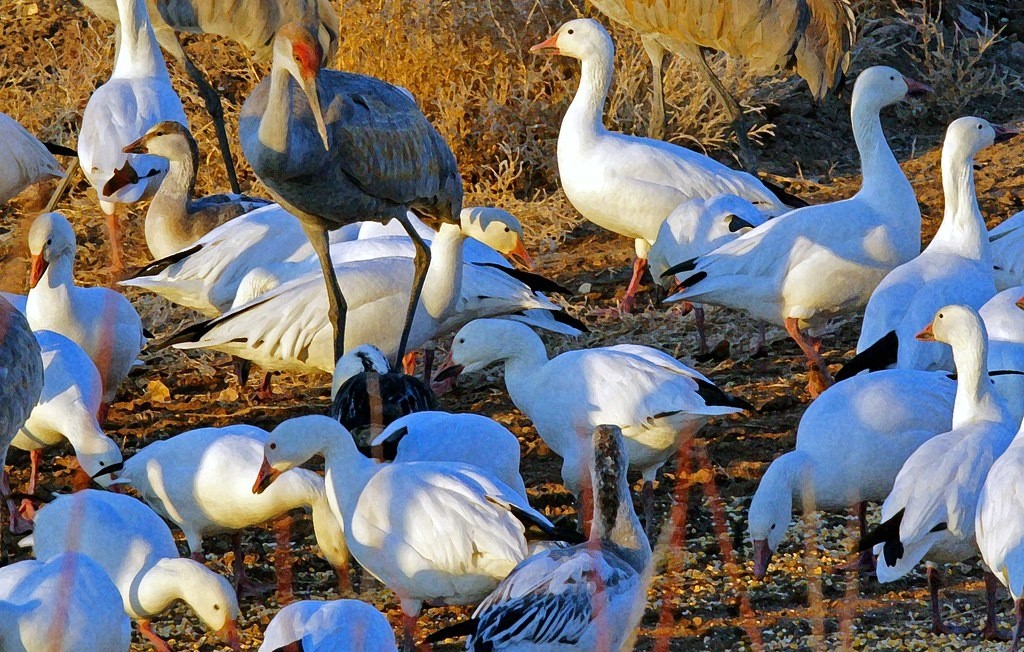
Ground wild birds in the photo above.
[75,0,341,199]
[237,17,465,422]
[584,0,858,190]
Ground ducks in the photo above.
[1,0,1023,651]
